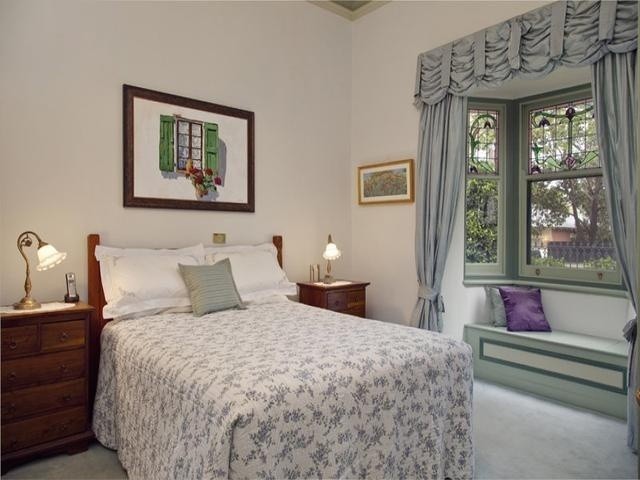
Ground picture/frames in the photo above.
[122,85,255,213]
[356,158,414,206]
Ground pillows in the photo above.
[179,254,245,318]
[497,285,556,333]
[95,243,210,319]
[208,242,299,304]
[484,283,508,330]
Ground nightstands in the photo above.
[297,276,371,318]
[2,292,94,471]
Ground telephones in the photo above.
[65,272,79,303]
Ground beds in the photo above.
[88,234,474,480]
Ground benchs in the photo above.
[460,320,633,422]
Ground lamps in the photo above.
[322,233,341,283]
[7,229,67,309]
